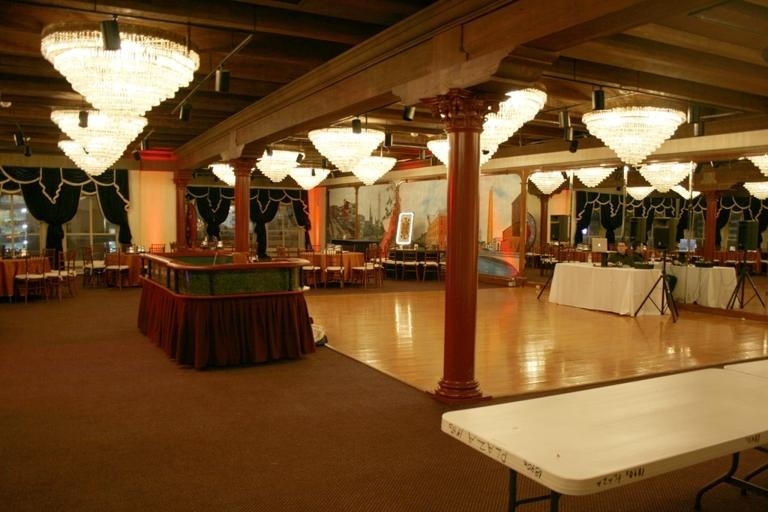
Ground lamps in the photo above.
[131,130,155,163]
[58,139,127,177]
[48,106,148,160]
[742,181,768,201]
[41,23,200,123]
[211,99,418,196]
[745,155,768,177]
[172,36,258,124]
[425,88,702,201]
[79,100,87,127]
[102,16,122,53]
[12,120,33,157]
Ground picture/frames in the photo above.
[396,212,414,245]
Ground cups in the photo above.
[413,243,418,250]
[698,256,704,262]
[124,243,145,254]
[3,248,28,258]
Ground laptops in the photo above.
[591,238,619,253]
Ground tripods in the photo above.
[633,260,679,322]
[725,259,767,312]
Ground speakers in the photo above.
[629,217,647,242]
[725,219,760,252]
[652,216,675,250]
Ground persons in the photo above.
[607,241,634,268]
[632,241,645,261]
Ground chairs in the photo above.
[278,242,447,291]
[0,244,166,306]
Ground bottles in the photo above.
[651,252,656,263]
[587,252,592,266]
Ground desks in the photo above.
[524,241,760,317]
